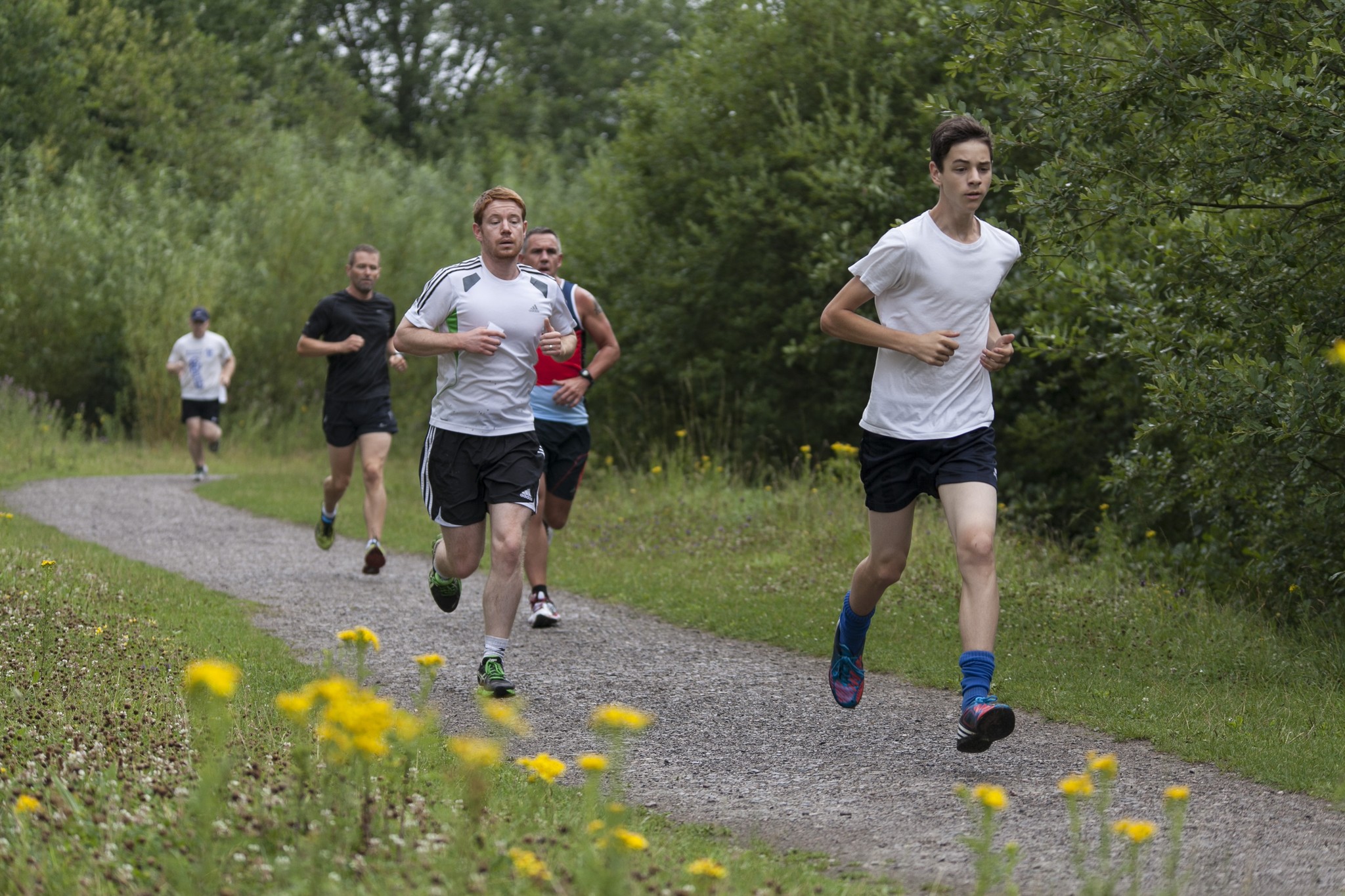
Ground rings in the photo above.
[549,344,552,350]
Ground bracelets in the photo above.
[389,352,401,357]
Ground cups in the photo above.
[485,321,505,343]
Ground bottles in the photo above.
[218,383,228,403]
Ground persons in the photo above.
[293,242,408,576]
[503,226,620,627]
[813,113,1021,755]
[165,308,237,483]
[390,183,578,691]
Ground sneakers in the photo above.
[828,617,866,709]
[208,439,220,452]
[362,542,387,575]
[955,694,1016,753]
[476,654,517,698]
[193,462,208,482]
[528,589,561,629]
[428,533,462,613]
[315,499,338,550]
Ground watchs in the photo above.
[579,368,594,385]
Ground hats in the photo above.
[190,307,210,323]
